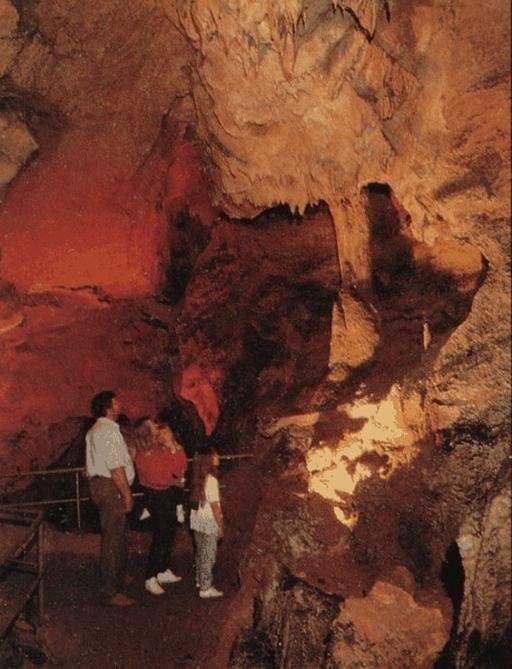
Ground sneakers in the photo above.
[175,503,186,524]
[157,568,183,584]
[144,575,166,596]
[199,585,224,599]
[138,507,151,521]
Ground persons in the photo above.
[84,390,136,608]
[139,424,188,523]
[186,446,224,598]
[133,415,188,596]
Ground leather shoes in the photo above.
[103,592,137,606]
[125,574,134,584]
